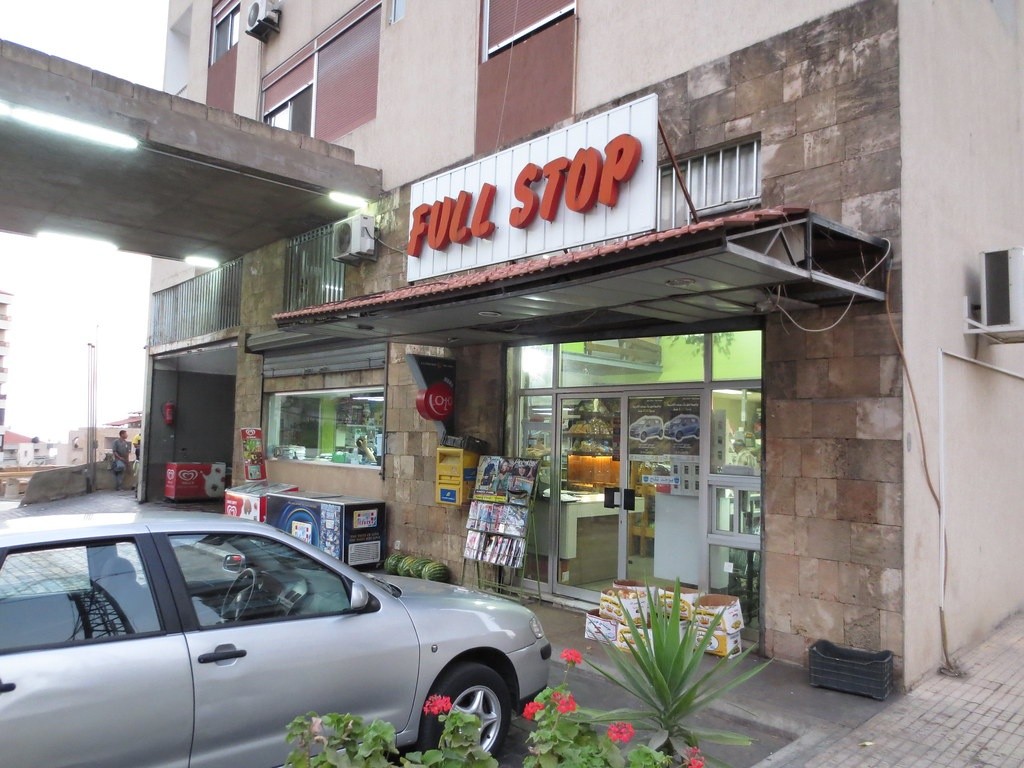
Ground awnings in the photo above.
[271,203,895,344]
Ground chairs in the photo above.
[99,557,161,631]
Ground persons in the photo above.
[112,430,129,492]
[356,435,377,464]
[478,457,531,493]
[131,425,141,490]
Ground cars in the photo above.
[0,518,553,768]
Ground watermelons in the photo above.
[384,552,448,582]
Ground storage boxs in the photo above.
[807,640,894,700]
[584,580,745,658]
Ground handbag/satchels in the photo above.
[113,460,125,472]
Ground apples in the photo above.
[604,589,646,600]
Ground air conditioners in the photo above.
[333,214,375,261]
[247,0,279,37]
[979,246,1024,344]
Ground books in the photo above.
[465,500,528,537]
[463,530,525,567]
[476,454,539,500]
